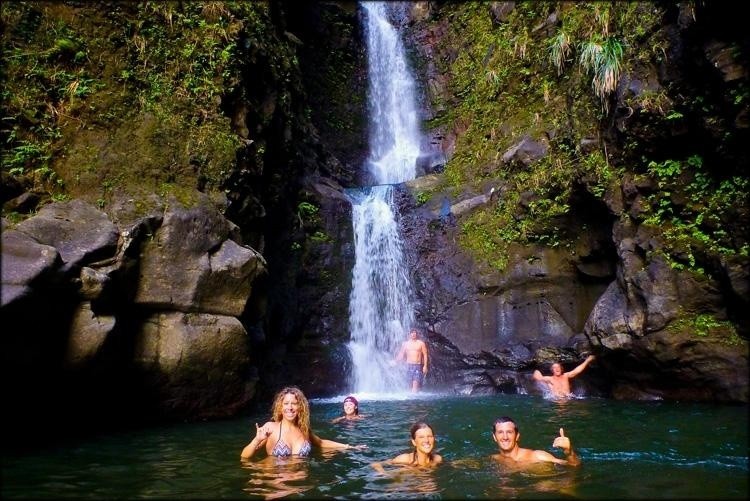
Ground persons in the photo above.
[330,396,366,424]
[240,385,370,460]
[533,353,596,404]
[488,416,582,501]
[396,329,428,394]
[371,421,482,496]
